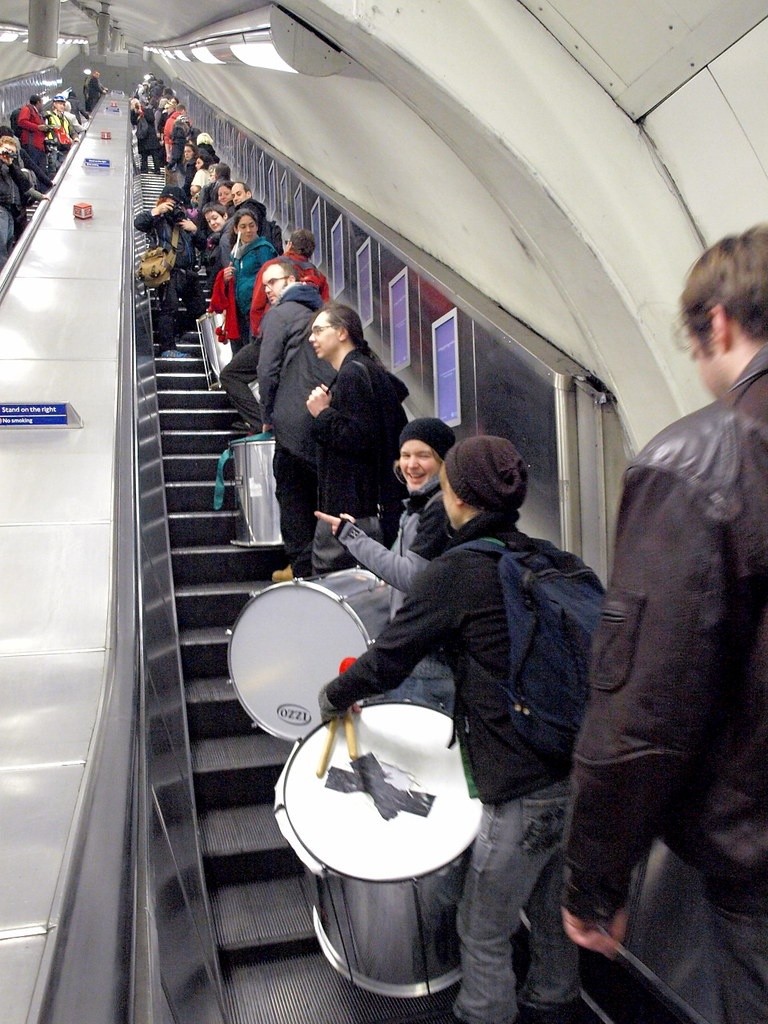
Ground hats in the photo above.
[400,417,456,460]
[159,185,186,203]
[445,434,528,512]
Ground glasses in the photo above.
[263,276,287,286]
[285,239,290,245]
[312,325,333,336]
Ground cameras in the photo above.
[166,200,186,224]
[5,152,18,158]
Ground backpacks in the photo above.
[10,104,33,139]
[442,535,607,758]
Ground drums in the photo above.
[227,436,286,548]
[196,310,234,391]
[226,563,395,745]
[272,696,484,999]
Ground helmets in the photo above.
[53,96,66,102]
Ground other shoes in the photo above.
[271,564,297,581]
[161,349,192,358]
[231,421,251,430]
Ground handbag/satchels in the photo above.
[16,149,38,206]
[137,246,176,287]
[136,115,149,140]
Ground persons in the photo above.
[132,184,208,358]
[130,71,459,722]
[563,225,768,1024]
[0,70,108,268]
[260,258,341,583]
[17,93,49,177]
[304,305,409,578]
[317,437,608,1024]
